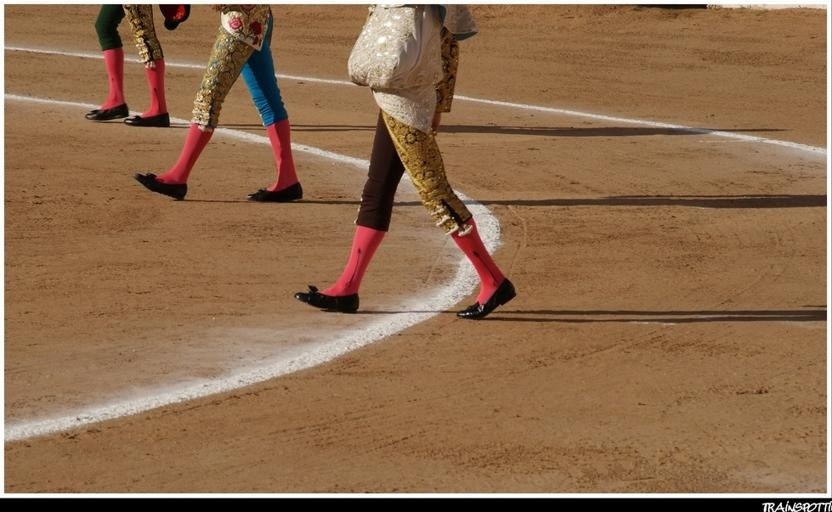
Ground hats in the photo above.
[160,4,190,29]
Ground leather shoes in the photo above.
[248,182,302,200]
[124,111,169,127]
[86,104,128,119]
[134,173,187,199]
[294,286,359,312]
[458,278,516,319]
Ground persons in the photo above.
[133,4,302,203]
[293,4,516,320]
[84,4,170,128]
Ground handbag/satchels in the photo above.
[347,7,444,88]
